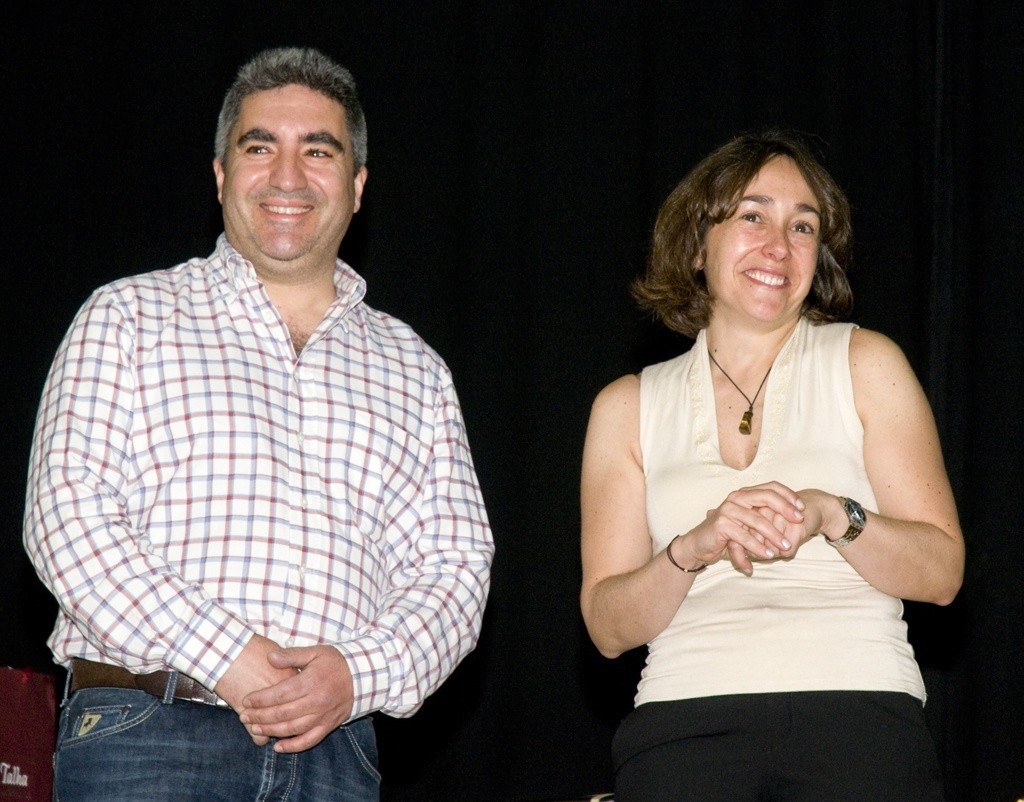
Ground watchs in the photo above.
[825,496,865,548]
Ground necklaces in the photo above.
[708,350,771,433]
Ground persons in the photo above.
[580,133,964,802]
[21,48,495,802]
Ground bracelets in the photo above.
[667,535,706,572]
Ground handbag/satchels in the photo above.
[0,667,56,802]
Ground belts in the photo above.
[68,656,232,708]
